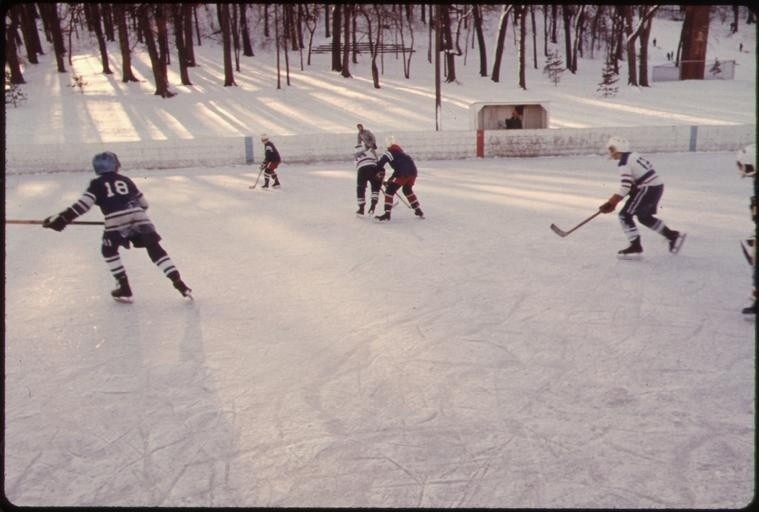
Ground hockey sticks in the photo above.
[381,180,412,210]
[249,168,262,189]
[549,210,601,238]
[379,186,399,208]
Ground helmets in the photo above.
[260,137,270,142]
[355,144,366,153]
[736,144,756,178]
[92,151,122,175]
[606,136,632,153]
[386,136,396,145]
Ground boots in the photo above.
[174,282,192,297]
[415,208,427,216]
[111,283,131,298]
[619,237,643,254]
[262,179,280,188]
[356,203,377,215]
[659,225,680,253]
[378,214,392,221]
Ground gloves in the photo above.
[43,216,69,231]
[599,193,623,214]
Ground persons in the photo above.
[355,144,386,215]
[505,111,522,129]
[355,123,378,159]
[736,144,758,316]
[259,136,281,188]
[42,150,192,299]
[375,144,423,220]
[597,135,679,254]
